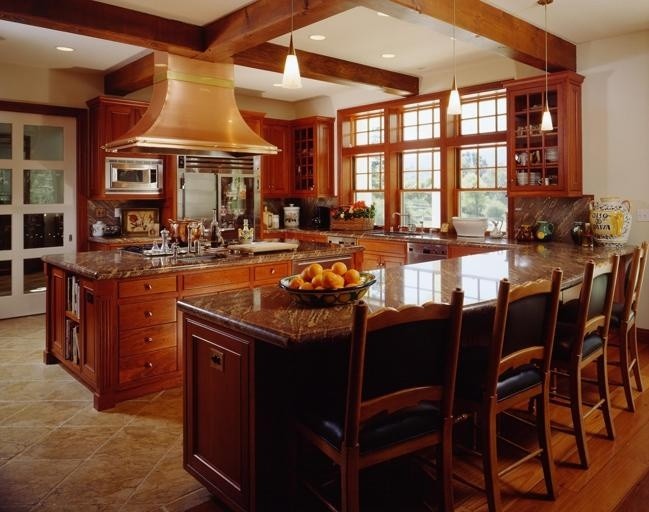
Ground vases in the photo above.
[331,218,374,230]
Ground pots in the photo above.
[283,204,301,227]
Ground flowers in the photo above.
[329,201,376,221]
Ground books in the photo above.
[64,274,82,367]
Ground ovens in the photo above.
[407,242,448,266]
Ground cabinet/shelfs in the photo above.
[260,116,335,197]
[503,70,584,198]
[84,96,170,157]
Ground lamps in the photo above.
[536,0,554,131]
[283,0,301,90]
[446,0,461,116]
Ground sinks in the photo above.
[371,232,421,237]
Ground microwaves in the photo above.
[104,158,164,195]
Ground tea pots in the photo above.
[536,220,554,242]
[92,221,106,236]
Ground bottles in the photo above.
[153,226,200,260]
[516,225,535,242]
[211,209,220,246]
[570,221,593,248]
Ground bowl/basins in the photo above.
[280,273,377,304]
[517,172,528,186]
[452,216,488,238]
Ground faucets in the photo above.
[392,212,411,232]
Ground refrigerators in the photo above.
[175,156,262,225]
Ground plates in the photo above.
[546,146,557,162]
[530,173,540,184]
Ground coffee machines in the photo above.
[311,206,331,230]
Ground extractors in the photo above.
[102,51,283,156]
